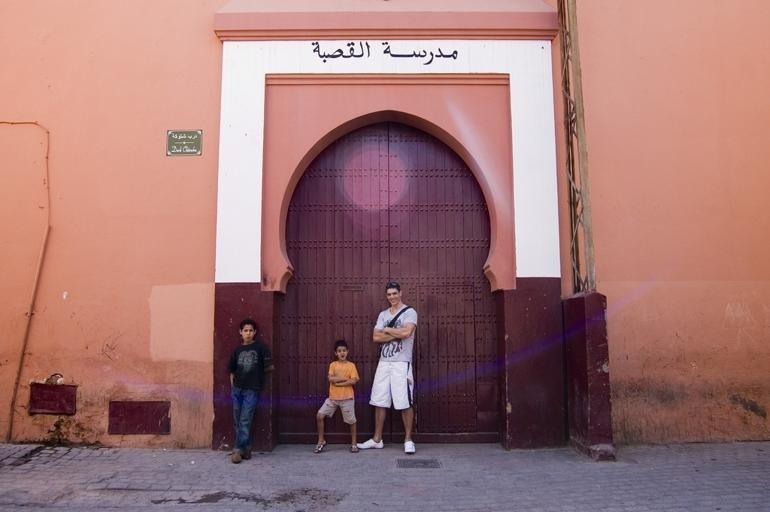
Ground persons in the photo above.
[355,281,419,454]
[224,319,273,464]
[312,339,359,453]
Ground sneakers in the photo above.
[402,440,415,454]
[356,438,385,450]
[231,448,251,462]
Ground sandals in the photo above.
[314,440,326,452]
[349,443,356,451]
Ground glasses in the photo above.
[385,281,400,287]
